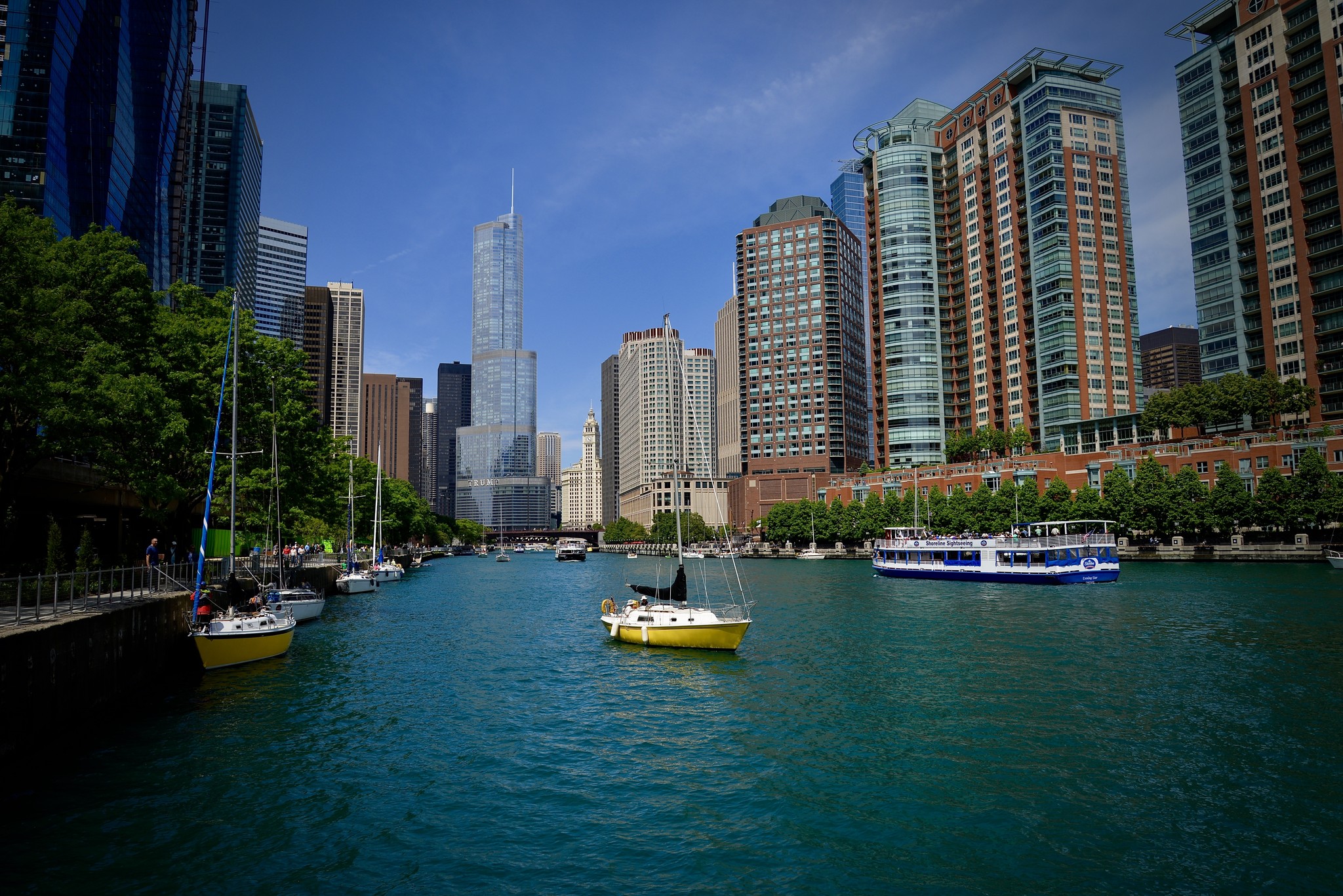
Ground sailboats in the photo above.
[795,511,826,560]
[367,439,405,584]
[336,424,380,595]
[186,324,298,672]
[495,502,511,562]
[713,507,746,559]
[259,423,328,625]
[682,500,704,559]
[600,312,760,653]
[460,518,488,558]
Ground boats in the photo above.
[1323,497,1343,570]
[627,551,637,559]
[871,466,1121,585]
[555,539,586,561]
[445,548,454,558]
[664,556,672,558]
[513,543,547,553]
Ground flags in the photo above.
[929,512,933,516]
[740,521,744,525]
[756,524,761,528]
[756,520,761,524]
[1081,530,1094,543]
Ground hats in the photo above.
[1037,526,1040,528]
[626,599,635,605]
[343,560,346,561]
[989,533,991,535]
[296,545,299,546]
[295,542,297,544]
[1006,532,1009,533]
[201,582,207,587]
[371,564,373,565]
[969,534,971,535]
[172,542,177,545]
[936,534,939,536]
[640,596,648,601]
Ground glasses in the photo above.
[152,541,157,542]
[643,599,646,600]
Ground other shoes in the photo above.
[155,589,164,591]
[148,589,155,592]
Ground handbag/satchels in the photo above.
[186,565,194,573]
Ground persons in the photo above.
[1149,536,1161,547]
[386,558,397,567]
[897,524,1070,547]
[346,544,373,552]
[873,551,881,560]
[371,562,380,571]
[383,544,425,555]
[183,546,199,584]
[639,596,651,607]
[625,599,635,617]
[854,480,866,487]
[163,542,184,584]
[262,542,325,567]
[337,558,355,580]
[146,538,164,593]
[301,577,314,600]
[735,545,744,553]
[434,545,439,548]
[191,582,212,634]
[714,548,725,555]
[1020,464,1022,471]
